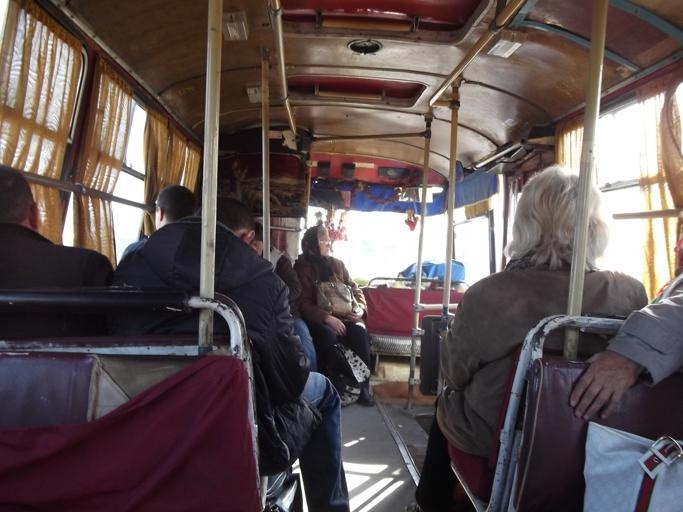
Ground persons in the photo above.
[120,183,196,259]
[108,199,349,512]
[0,162,114,338]
[404,164,647,512]
[293,226,375,406]
[569,78,683,424]
[250,223,317,370]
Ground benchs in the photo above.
[365,277,471,376]
[0,283,309,511]
[448,315,682,511]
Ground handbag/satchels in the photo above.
[316,280,358,322]
[581,418,682,511]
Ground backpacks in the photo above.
[328,342,370,407]
